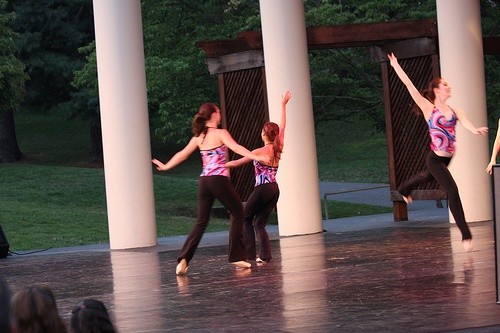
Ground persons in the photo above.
[152,104,271,276]
[11,287,68,333]
[68,299,115,333]
[486,117,500,174]
[387,52,489,250]
[212,89,293,265]
